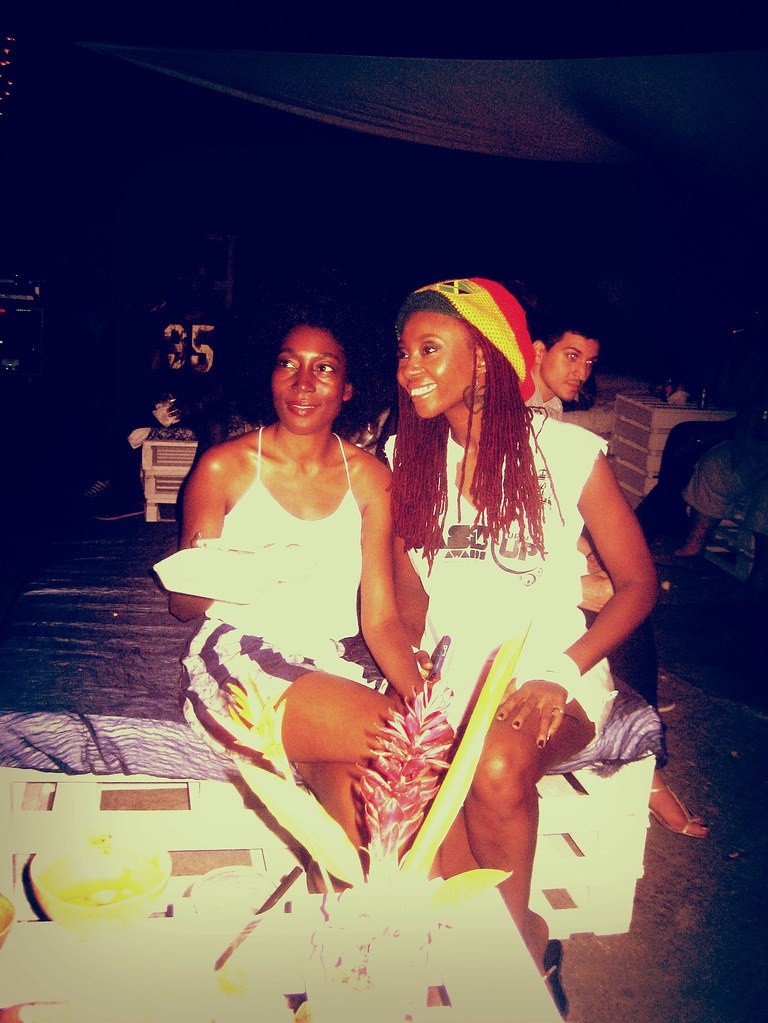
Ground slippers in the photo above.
[542,938,569,1015]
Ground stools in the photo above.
[514,675,665,937]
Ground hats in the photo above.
[415,276,536,402]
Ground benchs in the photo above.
[140,441,202,523]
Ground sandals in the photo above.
[645,784,708,839]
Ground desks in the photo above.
[0,766,567,1023]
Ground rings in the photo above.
[554,707,565,714]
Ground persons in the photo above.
[128,390,199,448]
[169,299,422,853]
[519,304,768,841]
[391,275,658,1014]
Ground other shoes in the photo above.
[655,694,676,712]
